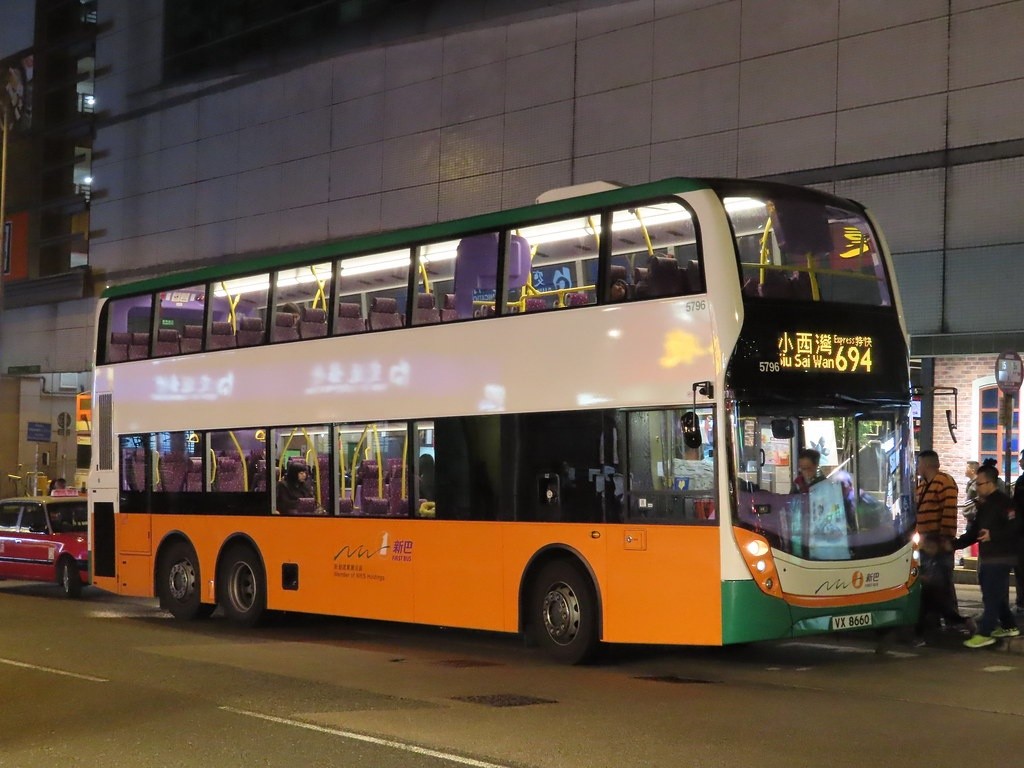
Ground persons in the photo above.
[835,469,890,532]
[277,462,314,512]
[610,279,631,300]
[420,455,432,498]
[48,478,65,495]
[913,450,963,646]
[50,511,59,520]
[788,449,828,497]
[957,449,1024,648]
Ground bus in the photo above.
[86,169,958,667]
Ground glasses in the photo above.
[975,480,993,486]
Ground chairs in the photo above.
[121,446,411,517]
[106,258,704,365]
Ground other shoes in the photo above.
[912,637,927,647]
[942,622,965,632]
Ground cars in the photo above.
[0,489,91,596]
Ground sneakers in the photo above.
[963,634,996,648]
[990,625,1020,638]
[1010,604,1024,616]
